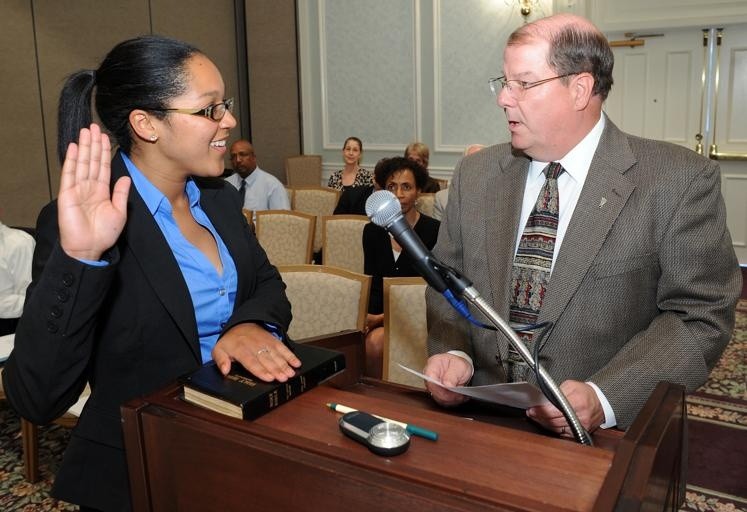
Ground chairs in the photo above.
[284,154,324,186]
[321,213,374,279]
[380,274,431,392]
[291,186,342,257]
[0,224,37,401]
[273,266,374,340]
[242,208,253,228]
[252,209,317,271]
[21,384,93,485]
[414,193,435,217]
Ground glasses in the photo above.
[143,97,234,122]
[489,72,584,99]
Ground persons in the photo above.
[327,137,374,189]
[225,140,292,234]
[404,143,441,193]
[1,35,301,511]
[313,184,383,265]
[1,221,36,363]
[433,144,487,221]
[561,426,567,434]
[345,157,440,365]
[424,13,742,512]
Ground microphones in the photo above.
[365,190,471,319]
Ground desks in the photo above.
[122,330,688,512]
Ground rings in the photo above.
[254,347,270,355]
[431,392,433,396]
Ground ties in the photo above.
[238,181,246,208]
[506,163,567,385]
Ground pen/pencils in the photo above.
[325,402,439,441]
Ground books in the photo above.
[177,344,346,420]
